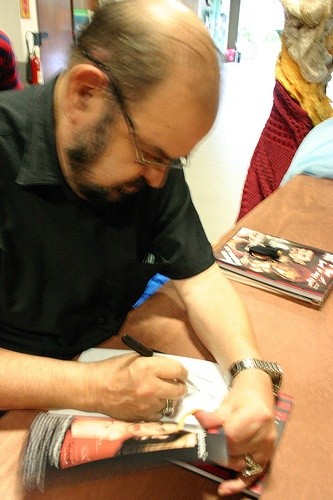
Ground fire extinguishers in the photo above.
[25,32,40,86]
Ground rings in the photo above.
[241,455,263,477]
[161,399,176,417]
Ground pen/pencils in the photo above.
[121,334,198,390]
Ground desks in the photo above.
[0,172,333,500]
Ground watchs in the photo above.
[224,359,284,397]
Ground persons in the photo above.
[0,0,283,496]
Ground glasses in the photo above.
[81,50,189,172]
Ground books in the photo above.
[14,346,295,499]
[213,226,333,308]
